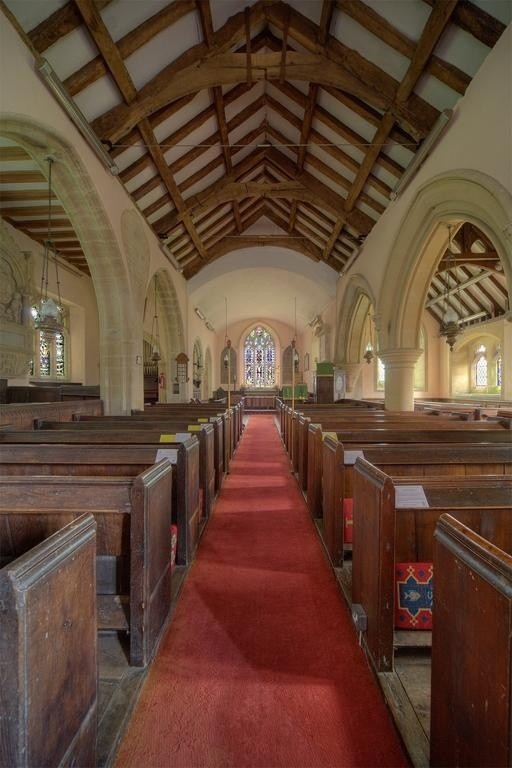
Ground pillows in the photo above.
[393,561,434,630]
[342,497,354,545]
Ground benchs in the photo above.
[274,396,512,768]
[0,383,245,768]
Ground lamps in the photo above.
[159,242,185,273]
[33,156,65,344]
[439,223,463,352]
[363,312,376,365]
[341,247,360,273]
[150,274,162,367]
[194,307,206,322]
[35,57,120,177]
[389,108,453,205]
[293,297,299,371]
[223,295,229,370]
